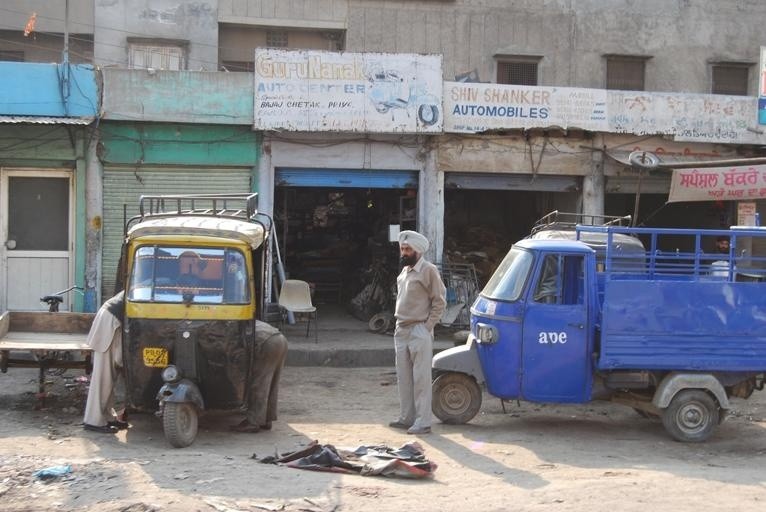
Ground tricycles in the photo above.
[1,286,97,405]
[110,193,273,447]
[363,64,439,125]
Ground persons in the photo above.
[82,273,172,434]
[224,317,288,434]
[387,228,448,435]
[707,237,739,278]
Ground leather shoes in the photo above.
[260,420,272,430]
[229,419,260,433]
[107,419,127,429]
[84,423,118,433]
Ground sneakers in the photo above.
[389,421,412,429]
[407,424,431,434]
